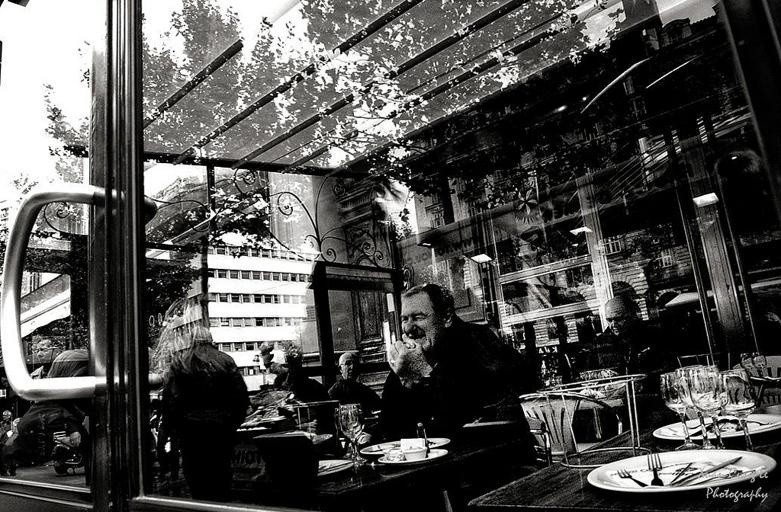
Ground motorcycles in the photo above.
[50,429,84,475]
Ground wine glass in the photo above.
[580,368,613,392]
[660,365,757,452]
[334,402,367,470]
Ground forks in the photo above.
[647,453,664,487]
[617,469,648,488]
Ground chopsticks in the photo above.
[669,462,700,485]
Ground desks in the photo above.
[468,428,781,509]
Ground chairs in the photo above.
[516,392,623,466]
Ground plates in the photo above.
[652,414,781,441]
[317,437,451,477]
[587,450,776,494]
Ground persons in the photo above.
[158,324,255,505]
[374,283,549,448]
[24,339,61,385]
[328,347,383,404]
[591,292,680,377]
[1,410,22,479]
[49,345,94,485]
